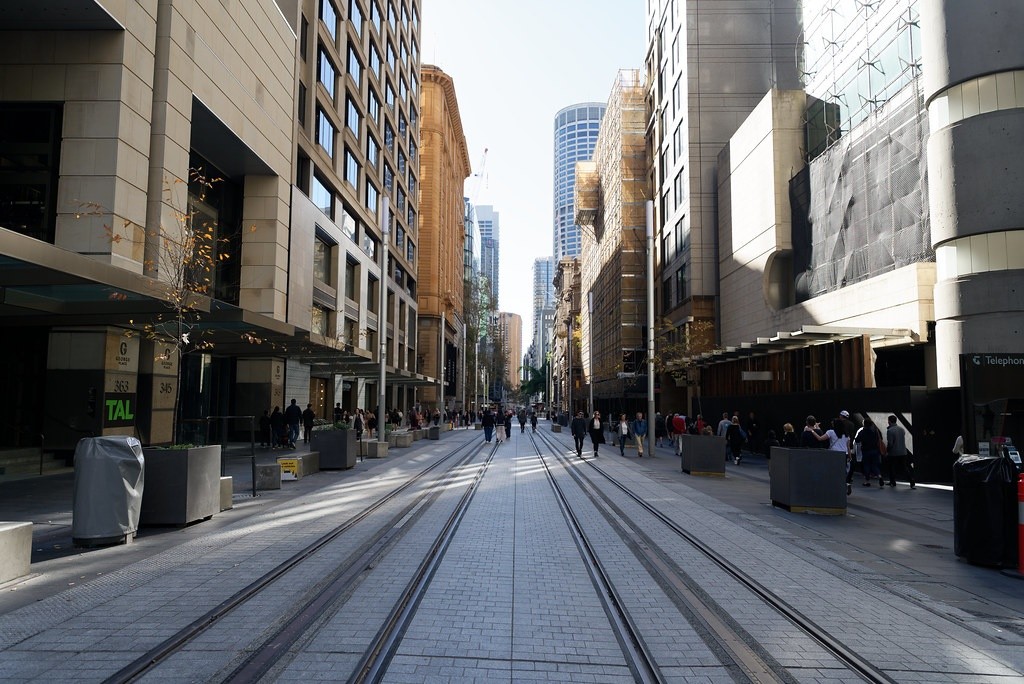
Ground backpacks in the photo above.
[409,411,415,420]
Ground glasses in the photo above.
[579,413,583,415]
[596,414,599,415]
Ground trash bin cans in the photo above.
[953,454,1019,562]
[71,436,144,543]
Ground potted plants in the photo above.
[644,316,726,476]
[309,421,358,471]
[141,443,221,527]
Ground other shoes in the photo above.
[655,443,663,448]
[675,453,683,456]
[622,453,624,456]
[577,449,581,457]
[862,481,871,486]
[260,442,296,451]
[594,452,599,457]
[879,483,884,489]
[846,482,851,496]
[521,431,524,433]
[486,440,503,443]
[734,457,740,465]
[638,452,642,457]
[908,484,915,489]
[886,482,896,486]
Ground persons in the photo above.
[546,411,558,424]
[260,399,315,450]
[766,410,917,496]
[571,410,587,457]
[589,411,605,457]
[612,412,647,457]
[333,400,527,444]
[655,410,760,465]
[530,412,538,433]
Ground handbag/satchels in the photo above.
[877,429,886,455]
[406,421,409,426]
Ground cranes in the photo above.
[471,146,490,208]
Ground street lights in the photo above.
[557,346,560,412]
[475,343,478,422]
[377,196,390,443]
[440,311,445,425]
[646,199,655,458]
[588,291,593,419]
[569,324,572,428]
[463,324,467,415]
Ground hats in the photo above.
[840,410,849,418]
